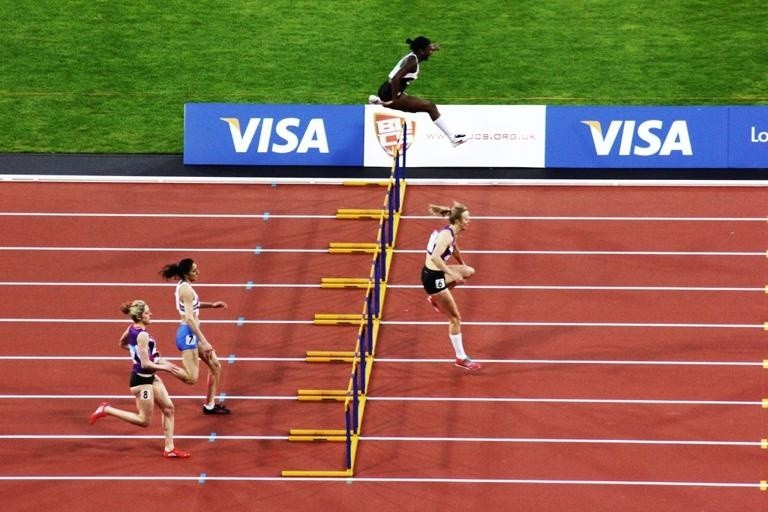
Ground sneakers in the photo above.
[427,296,443,315]
[452,133,473,147]
[203,404,231,415]
[368,95,380,104]
[455,355,481,371]
[88,402,111,426]
[163,447,191,458]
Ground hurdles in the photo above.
[289,355,366,435]
[319,242,394,282]
[304,298,381,356]
[335,123,406,214]
[329,182,401,248]
[281,394,359,476]
[314,278,387,320]
[297,322,374,396]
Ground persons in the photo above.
[153,258,233,414]
[368,35,473,147]
[89,300,191,459]
[419,199,482,373]
